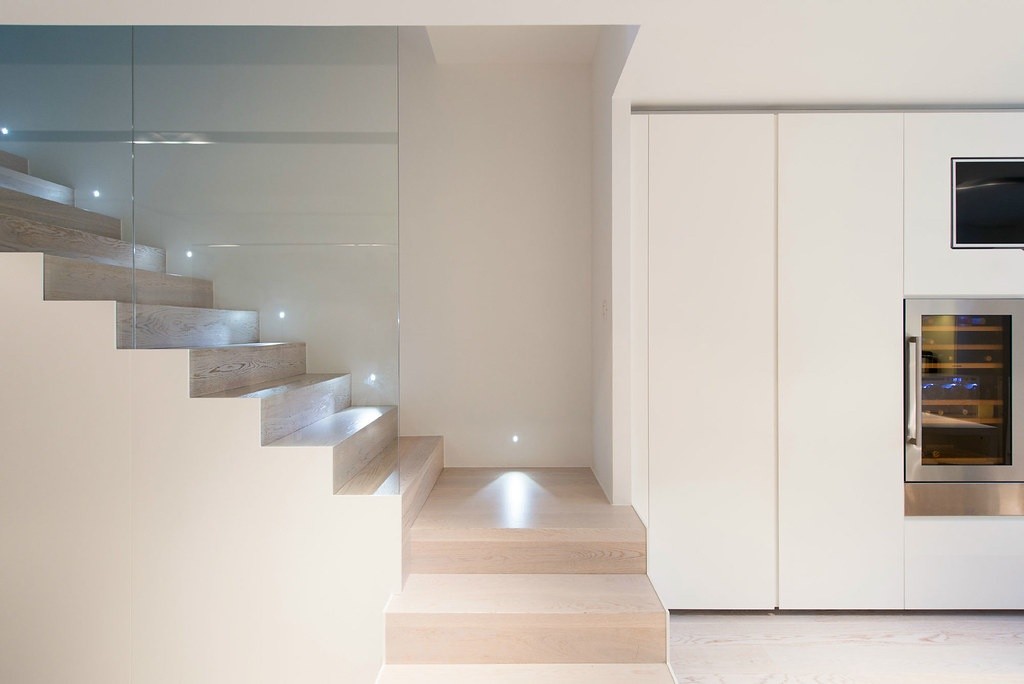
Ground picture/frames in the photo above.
[950,157,1024,250]
